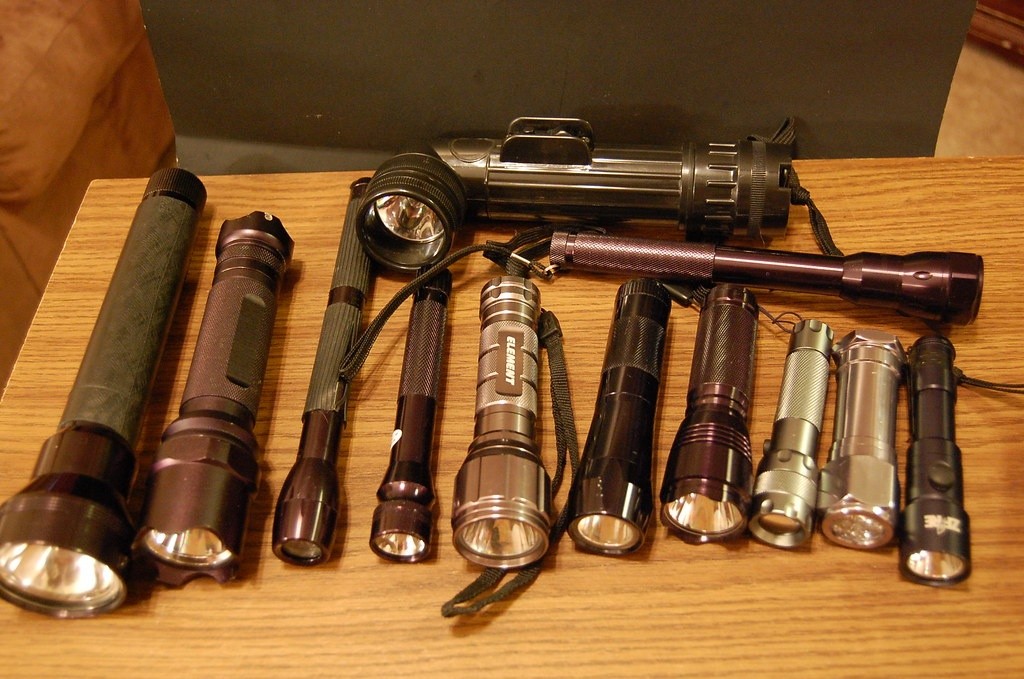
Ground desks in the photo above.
[1,155,1023,677]
